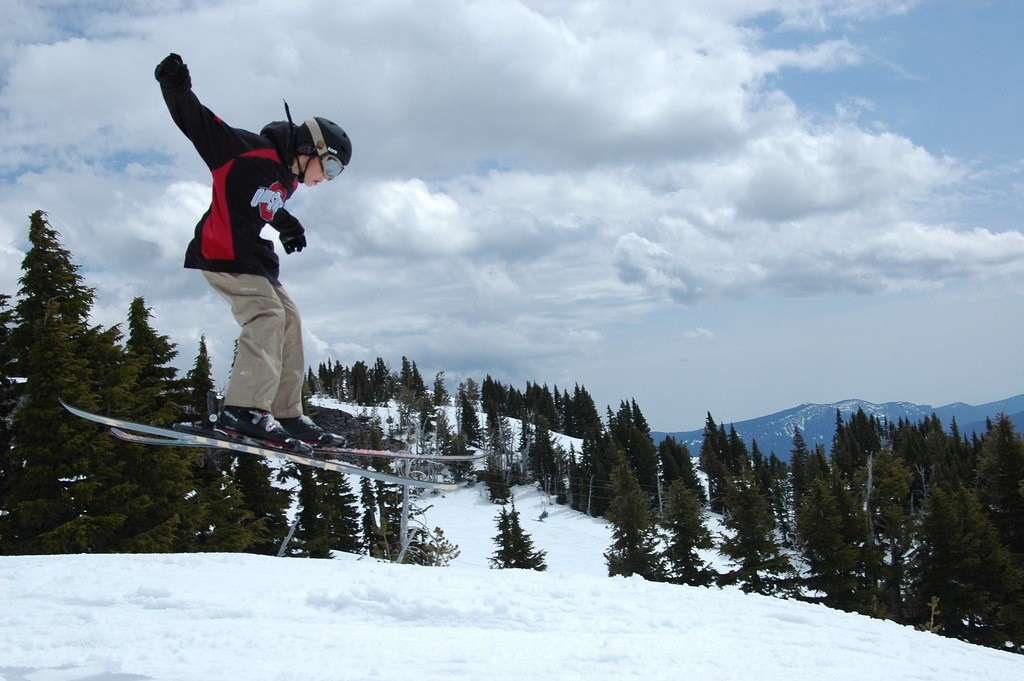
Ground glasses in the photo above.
[320,154,344,181]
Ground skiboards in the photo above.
[57,396,493,490]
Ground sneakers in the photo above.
[277,416,347,448]
[214,405,313,457]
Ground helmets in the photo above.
[298,117,352,165]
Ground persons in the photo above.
[154,50,355,453]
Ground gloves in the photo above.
[279,226,307,255]
[155,52,192,93]
